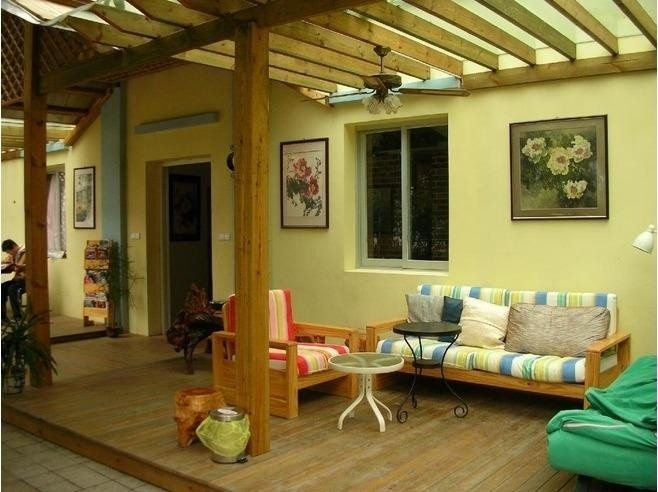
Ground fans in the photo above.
[298,44,471,116]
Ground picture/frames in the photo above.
[277,136,330,229]
[72,166,96,230]
[507,112,611,221]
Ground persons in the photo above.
[1,239,26,326]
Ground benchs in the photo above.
[366,281,629,416]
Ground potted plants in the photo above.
[99,238,135,336]
[2,306,57,391]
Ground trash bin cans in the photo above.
[209,406,248,464]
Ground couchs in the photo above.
[547,352,656,490]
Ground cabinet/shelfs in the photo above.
[80,237,114,327]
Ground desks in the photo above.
[326,348,406,432]
[391,319,471,423]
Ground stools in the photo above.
[168,385,224,447]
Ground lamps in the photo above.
[628,220,657,254]
[360,92,402,114]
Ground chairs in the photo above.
[169,280,220,375]
[211,287,360,420]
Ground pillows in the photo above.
[454,296,509,351]
[434,294,463,344]
[403,291,443,343]
[506,301,609,357]
